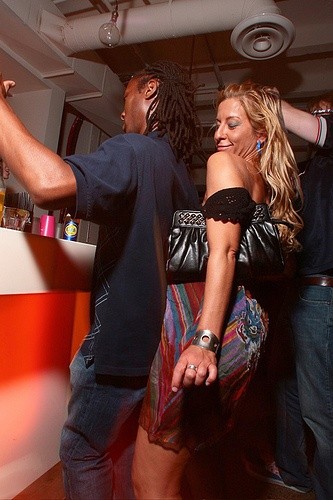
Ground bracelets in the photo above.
[191,329,220,354]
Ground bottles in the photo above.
[64,208,80,241]
[0,155,6,227]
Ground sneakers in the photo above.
[248,461,307,494]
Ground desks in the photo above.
[0,226,99,500]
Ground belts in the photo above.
[295,277,333,287]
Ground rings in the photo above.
[187,365,198,372]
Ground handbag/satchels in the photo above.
[165,203,294,284]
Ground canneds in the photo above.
[62,220,78,242]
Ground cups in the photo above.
[33,217,42,235]
[55,222,63,238]
[41,215,56,237]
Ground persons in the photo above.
[0,61,333,500]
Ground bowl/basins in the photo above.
[3,206,30,231]
